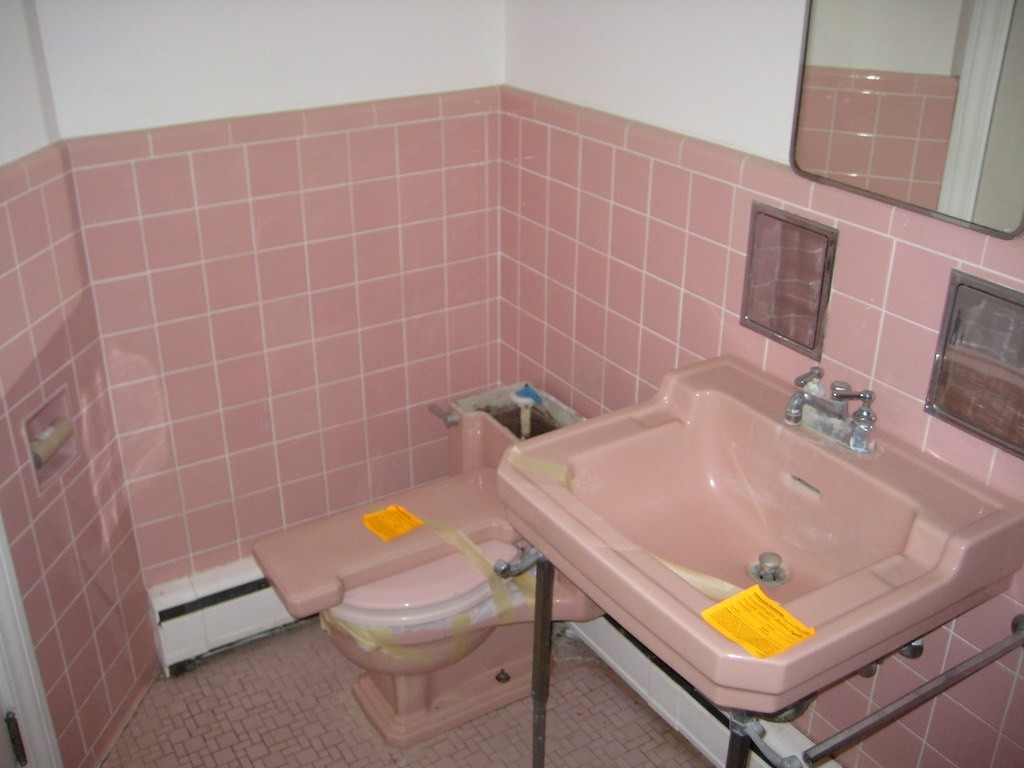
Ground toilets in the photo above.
[251,382,606,751]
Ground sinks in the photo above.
[496,404,954,714]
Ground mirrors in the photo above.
[788,0,1024,239]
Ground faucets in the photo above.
[784,381,852,425]
